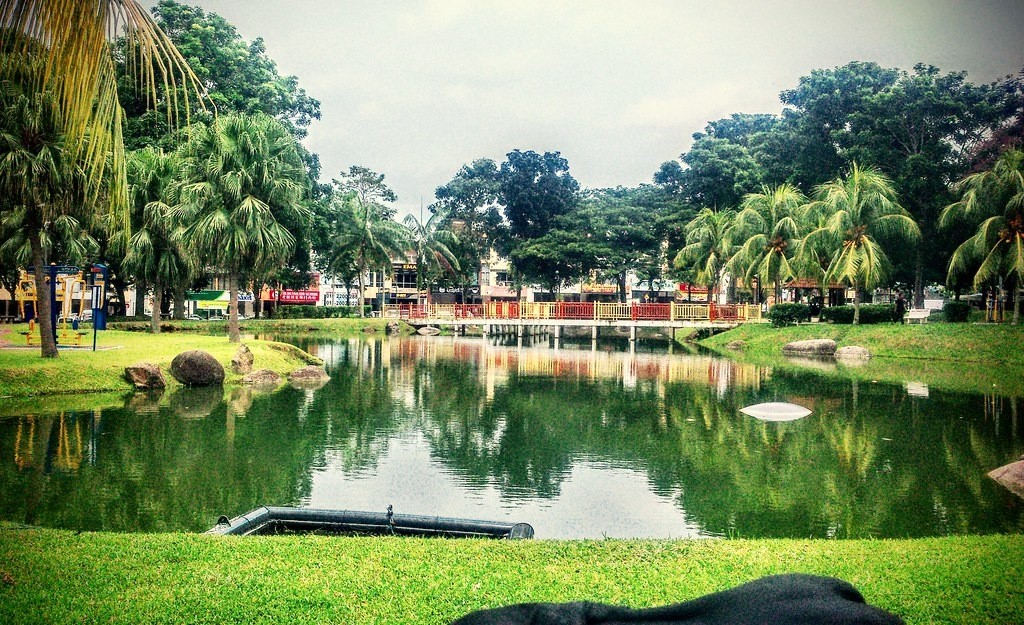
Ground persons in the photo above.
[893,292,906,324]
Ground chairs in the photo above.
[902,308,930,324]
[72,320,88,347]
[18,319,35,349]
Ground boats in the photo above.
[203,504,535,541]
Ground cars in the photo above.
[185,314,245,320]
[80,309,93,323]
[57,314,80,323]
